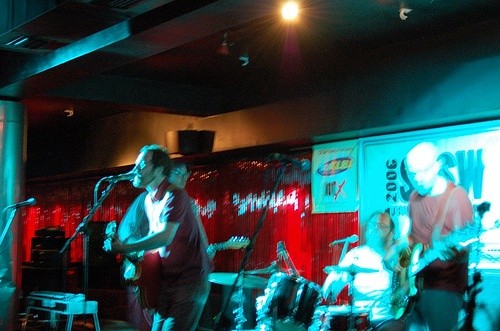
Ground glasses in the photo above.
[371,223,391,228]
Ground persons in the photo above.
[382,141,473,331]
[309,211,409,331]
[102,144,216,331]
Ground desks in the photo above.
[21,291,100,331]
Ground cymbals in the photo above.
[323,263,380,274]
[244,264,287,275]
[208,272,268,289]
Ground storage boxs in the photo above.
[22,263,84,290]
[32,238,70,267]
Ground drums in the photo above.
[261,272,322,331]
[308,306,372,331]
[254,295,269,331]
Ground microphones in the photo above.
[329,234,359,246]
[476,202,490,213]
[7,198,36,211]
[106,171,137,182]
[269,152,311,172]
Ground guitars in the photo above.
[105,220,145,282]
[390,220,482,321]
[276,241,300,276]
[206,236,250,252]
